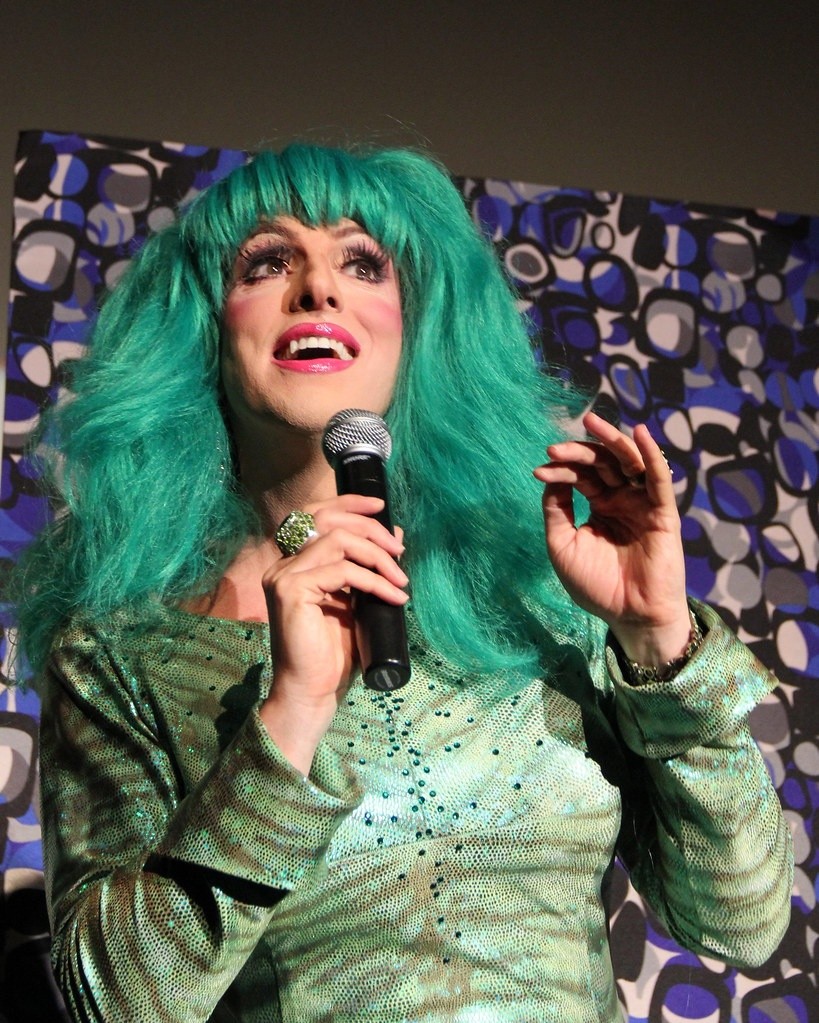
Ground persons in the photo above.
[13,138,792,1022]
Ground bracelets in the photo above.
[622,609,705,687]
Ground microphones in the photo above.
[321,408,411,692]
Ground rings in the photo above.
[272,510,317,558]
